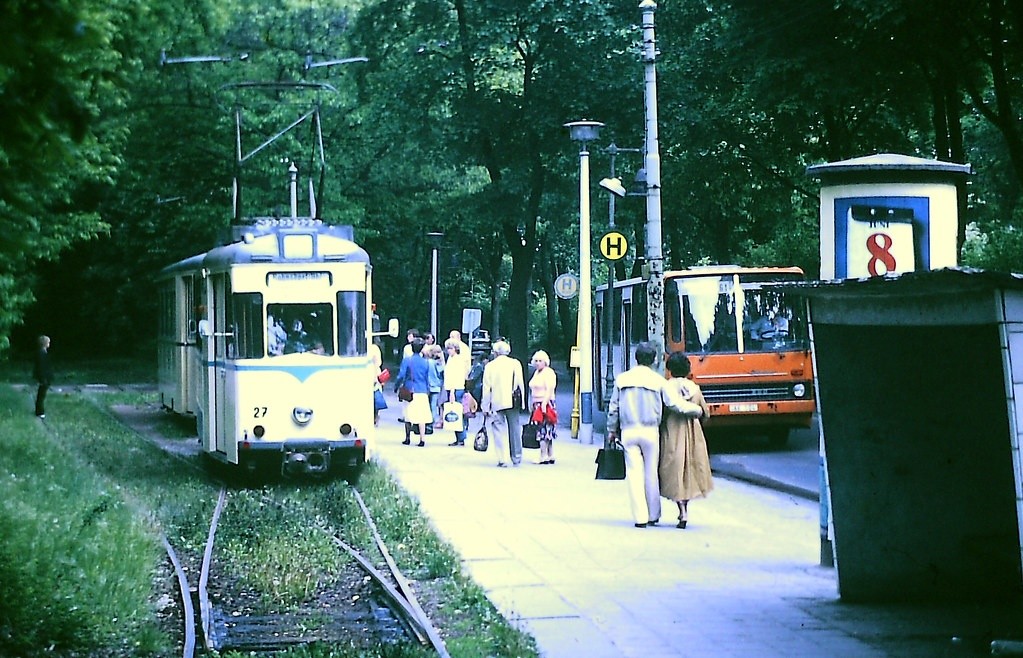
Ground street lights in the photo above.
[596,176,628,415]
[564,119,608,444]
[426,233,448,342]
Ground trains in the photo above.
[154,216,399,479]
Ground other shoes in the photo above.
[676,520,687,528]
[497,462,506,468]
[514,459,520,466]
[398,416,444,433]
[402,439,410,444]
[677,516,681,520]
[634,523,646,528]
[648,520,656,526]
[415,441,425,446]
[537,459,554,466]
[448,441,464,446]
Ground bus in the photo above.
[590,261,816,444]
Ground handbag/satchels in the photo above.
[397,387,413,401]
[462,391,477,418]
[511,386,522,410]
[443,401,463,432]
[474,415,488,451]
[595,438,625,480]
[374,389,388,411]
[521,413,540,449]
[377,369,390,383]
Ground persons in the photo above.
[659,351,712,530]
[33,336,51,418]
[606,344,703,528]
[267,315,324,354]
[372,330,493,447]
[528,350,555,465]
[481,342,524,467]
[750,302,788,339]
